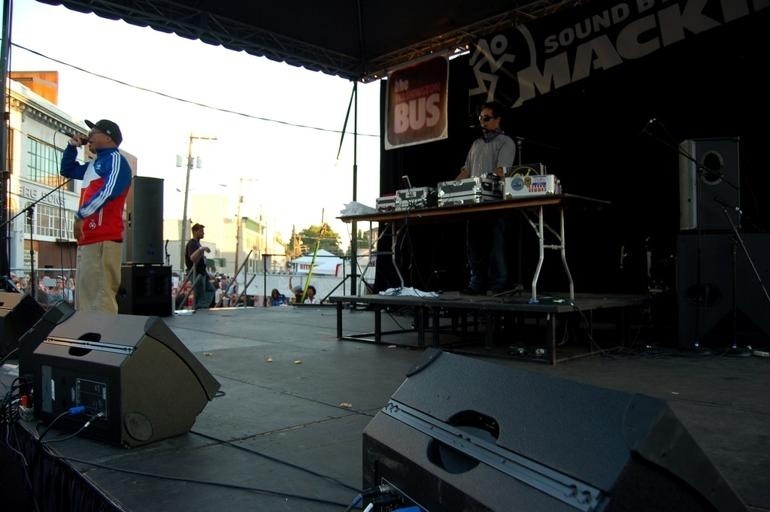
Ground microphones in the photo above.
[469,124,484,129]
[59,129,87,145]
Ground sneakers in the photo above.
[460,287,488,296]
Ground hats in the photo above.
[84,120,122,145]
[192,224,204,231]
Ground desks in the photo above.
[335,192,610,306]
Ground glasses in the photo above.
[478,115,496,121]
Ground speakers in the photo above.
[32,310,221,448]
[362,346,752,512]
[121,175,164,266]
[678,137,741,234]
[18,300,73,416]
[0,292,45,360]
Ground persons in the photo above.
[60,118,133,316]
[183,223,216,313]
[454,101,516,298]
[172,272,246,310]
[10,271,75,307]
[249,275,318,306]
